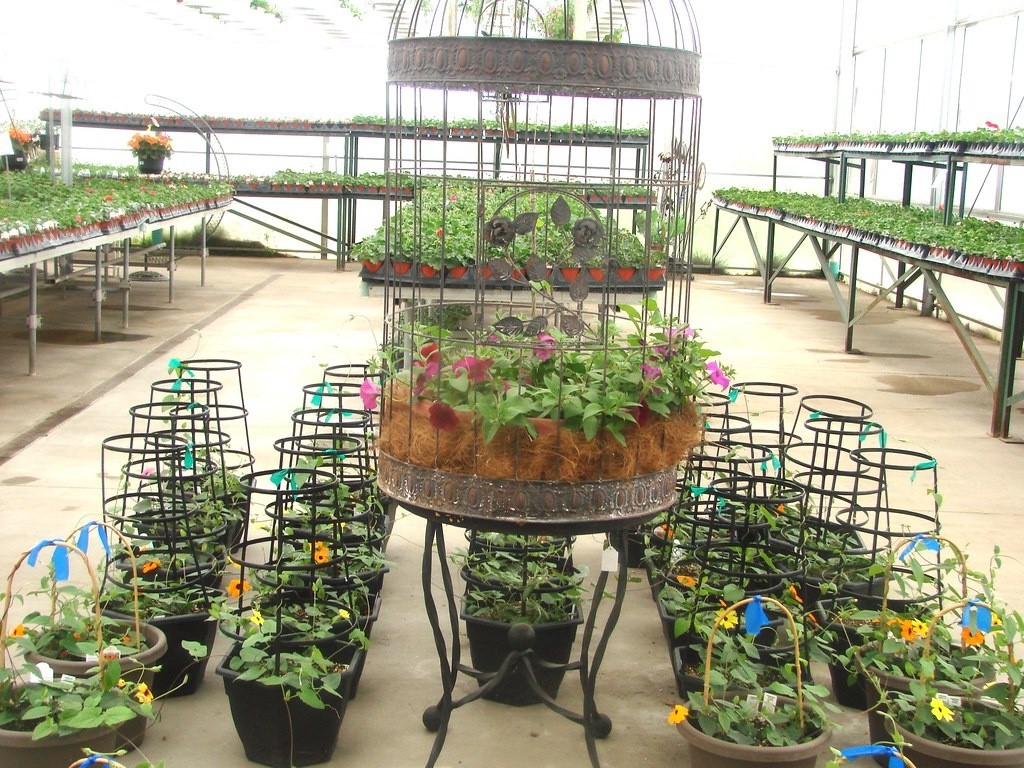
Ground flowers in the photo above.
[1,364,389,738]
[349,186,668,270]
[374,309,734,443]
[635,445,1023,747]
[0,126,274,239]
[459,0,623,44]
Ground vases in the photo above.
[1,194,234,264]
[0,482,399,768]
[250,184,257,189]
[38,133,61,150]
[362,260,666,283]
[608,526,1024,767]
[375,384,707,535]
[136,150,168,173]
[386,37,698,99]
[10,138,29,158]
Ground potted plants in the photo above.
[452,525,584,706]
[275,171,656,204]
[42,112,650,145]
[710,129,1024,277]
